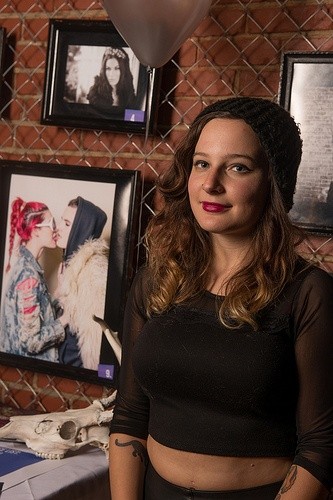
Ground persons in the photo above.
[52,196,109,371]
[0,195,70,370]
[108,97,333,500]
[86,47,136,109]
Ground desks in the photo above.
[0,416,112,500]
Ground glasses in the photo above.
[35,217,56,232]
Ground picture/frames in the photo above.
[276,50,333,238]
[0,160,143,390]
[40,17,158,136]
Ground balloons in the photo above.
[99,0,211,74]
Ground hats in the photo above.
[192,97,303,213]
